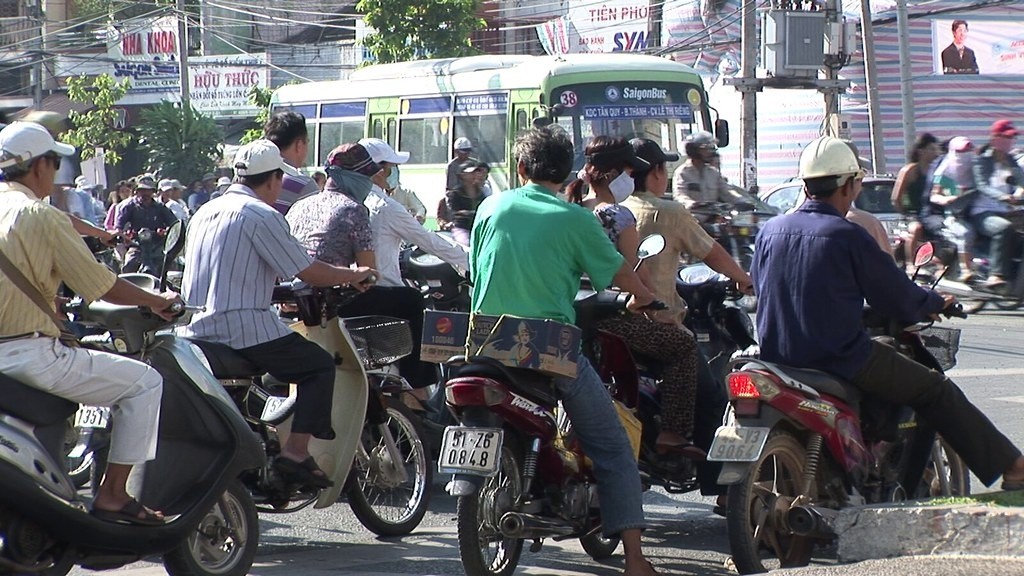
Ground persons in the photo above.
[0,120,186,525]
[24,171,232,298]
[565,133,709,462]
[265,111,492,416]
[469,123,659,576]
[889,119,1024,288]
[749,135,1024,490]
[180,140,380,488]
[619,137,754,497]
[672,131,746,225]
[942,19,980,75]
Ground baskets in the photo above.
[343,314,414,366]
[919,326,960,370]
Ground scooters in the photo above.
[59,181,789,509]
[899,175,1024,315]
[0,216,270,576]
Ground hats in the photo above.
[158,179,174,192]
[171,179,188,190]
[454,136,473,153]
[629,138,680,170]
[358,137,411,165]
[949,136,972,151]
[215,176,231,190]
[0,121,75,169]
[992,119,1019,137]
[136,175,157,189]
[202,173,217,182]
[324,143,382,190]
[585,142,652,170]
[844,140,872,169]
[455,160,481,177]
[74,175,93,190]
[233,139,299,177]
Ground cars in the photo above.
[757,170,915,253]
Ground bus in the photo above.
[267,52,731,233]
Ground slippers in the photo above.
[657,442,706,462]
[712,506,726,518]
[92,498,166,525]
[279,455,334,488]
[1000,479,1024,490]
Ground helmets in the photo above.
[796,136,860,180]
[686,132,719,159]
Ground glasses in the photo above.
[44,155,61,170]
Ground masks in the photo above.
[606,170,634,203]
[386,167,400,190]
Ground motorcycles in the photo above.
[440,233,672,576]
[165,271,434,537]
[705,240,969,576]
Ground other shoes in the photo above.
[987,277,1007,290]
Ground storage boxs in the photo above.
[420,310,583,379]
